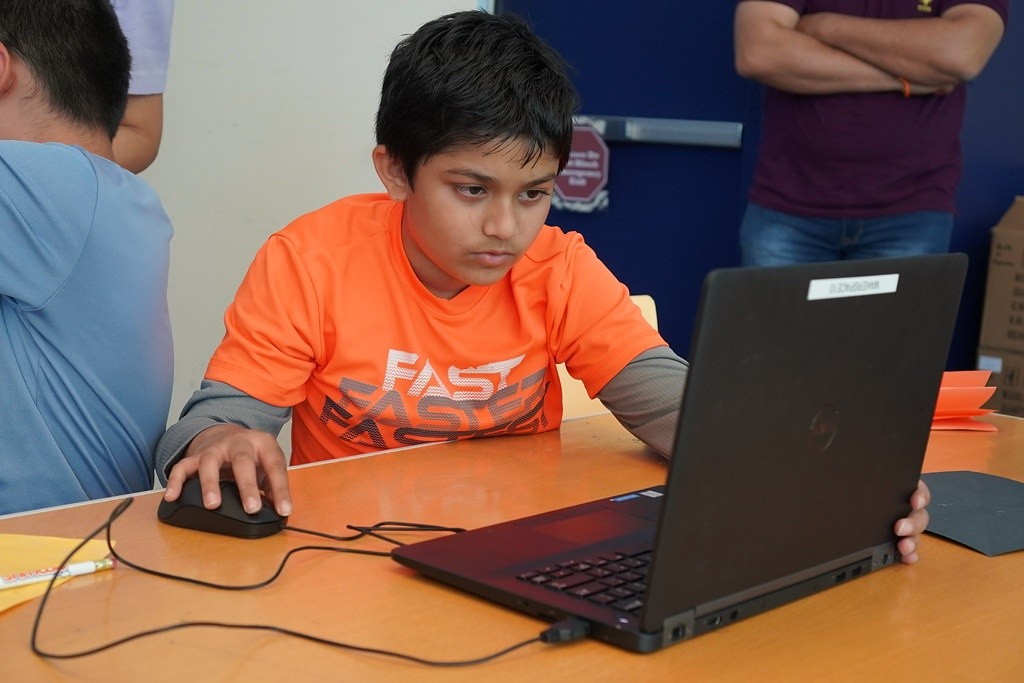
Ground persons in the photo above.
[155,10,932,566]
[733,0,1010,265]
[0,0,173,515]
[107,0,174,174]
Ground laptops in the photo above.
[392,252,972,654]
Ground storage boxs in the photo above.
[976,195,1024,419]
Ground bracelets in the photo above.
[900,77,910,96]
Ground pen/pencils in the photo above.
[0,559,118,590]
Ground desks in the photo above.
[0,411,1024,683]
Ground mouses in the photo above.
[157,477,289,539]
